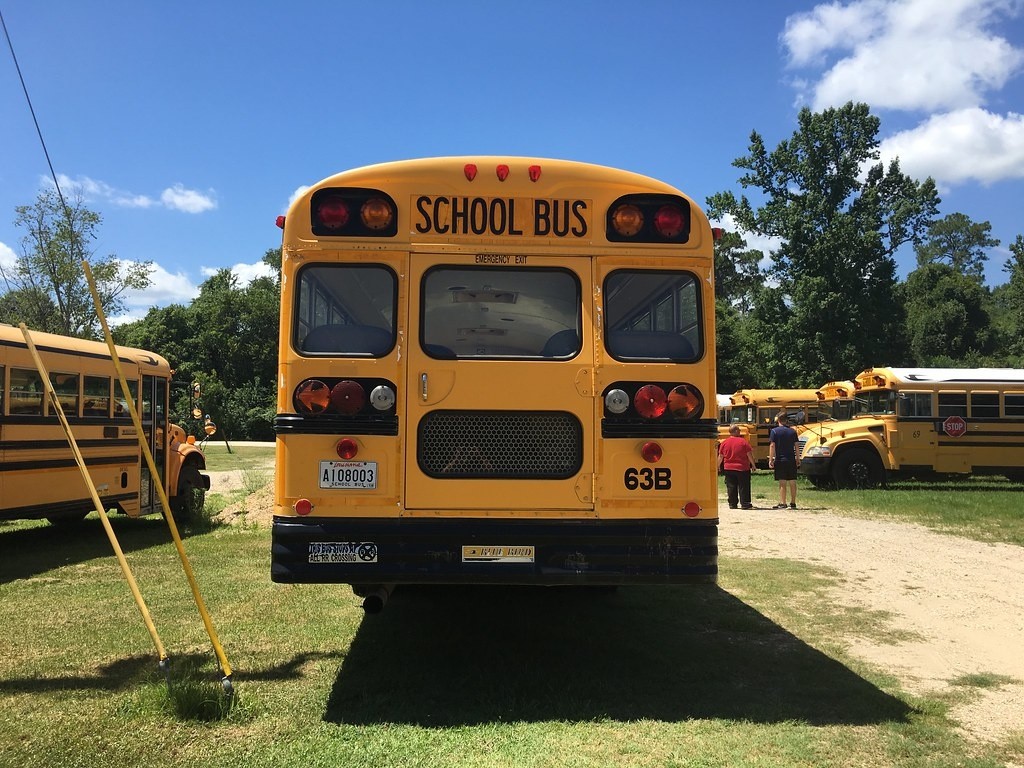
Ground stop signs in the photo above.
[943,416,967,438]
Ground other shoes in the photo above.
[730,506,738,509]
[742,507,757,510]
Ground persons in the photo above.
[769,411,801,510]
[717,424,759,510]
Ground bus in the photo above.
[791,367,1024,488]
[0,324,217,531]
[270,154,721,621]
[716,394,737,426]
[716,388,820,472]
[767,381,855,473]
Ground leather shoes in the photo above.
[790,503,796,507]
[773,504,787,508]
